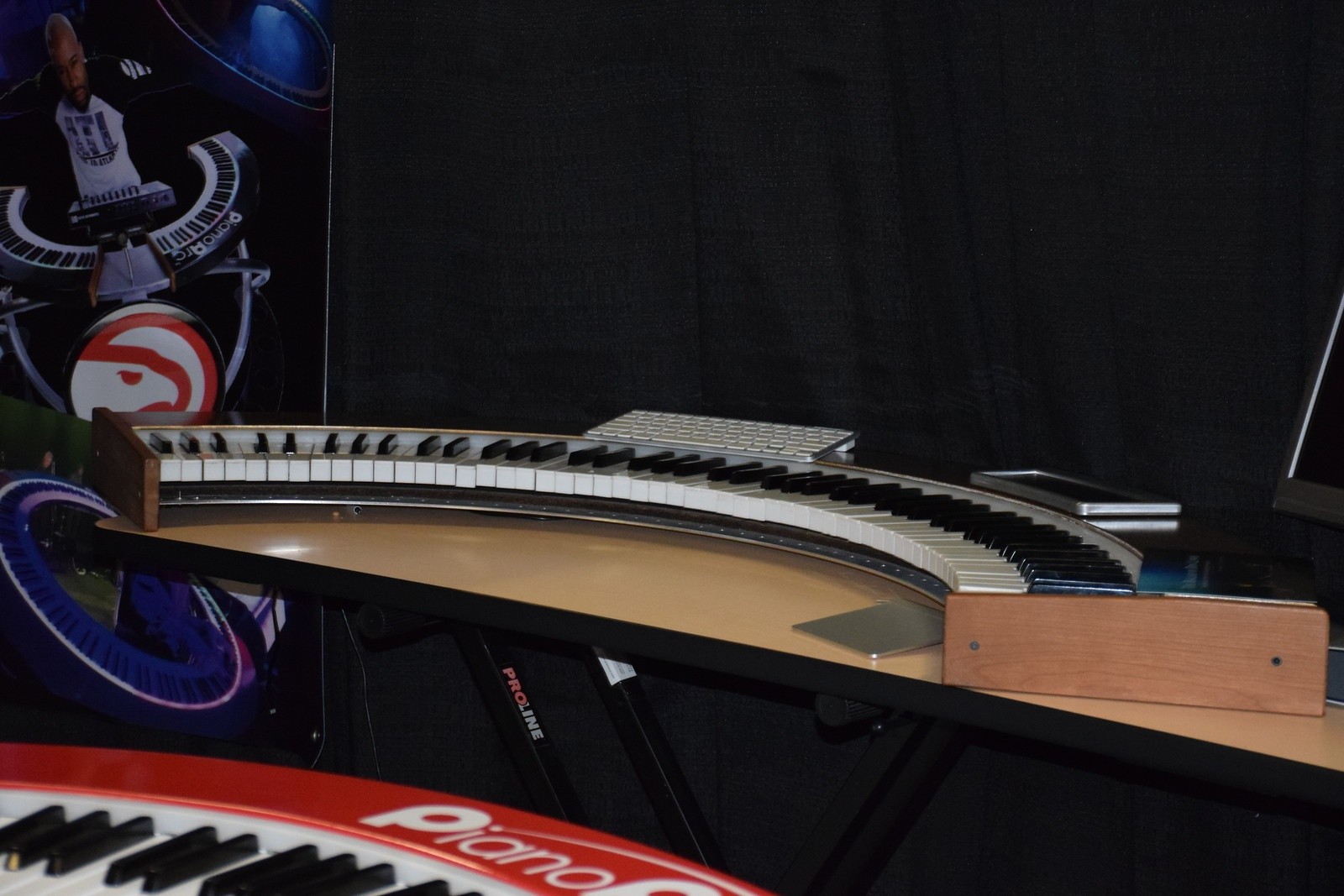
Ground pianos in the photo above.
[1,739,779,896]
[89,406,1332,719]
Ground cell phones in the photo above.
[971,468,1181,515]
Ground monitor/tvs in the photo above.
[1271,278,1343,533]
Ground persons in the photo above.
[30,451,103,575]
[0,12,222,246]
